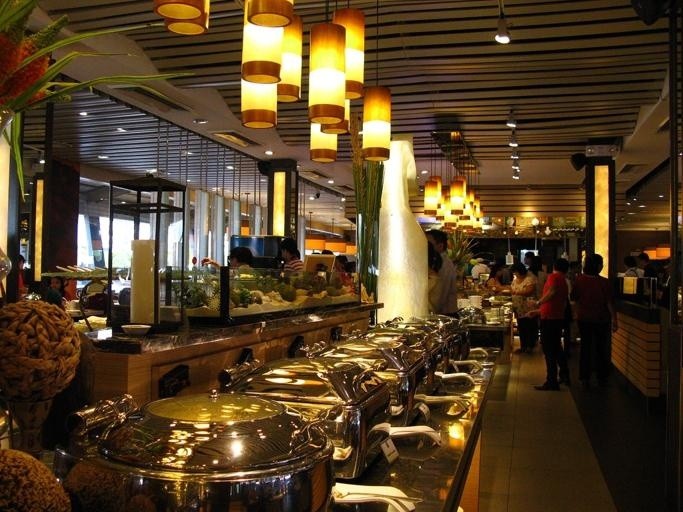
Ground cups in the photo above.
[484,305,511,325]
[456,296,483,311]
[73,288,83,299]
[465,273,489,290]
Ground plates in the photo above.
[74,315,107,331]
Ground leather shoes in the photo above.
[535,384,560,391]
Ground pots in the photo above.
[118,288,130,305]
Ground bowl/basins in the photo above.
[65,299,80,316]
[120,324,152,335]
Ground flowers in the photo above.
[0,0,197,205]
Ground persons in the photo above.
[51,276,71,300]
[5,255,27,303]
[472,251,658,354]
[425,229,459,319]
[526,259,570,391]
[570,254,618,389]
[201,238,357,276]
[428,241,446,313]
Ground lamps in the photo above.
[504,237,513,266]
[422,114,486,231]
[493,2,511,46]
[584,142,617,156]
[504,109,521,180]
[156,1,393,165]
[239,186,356,257]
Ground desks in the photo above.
[466,302,514,354]
[331,343,505,511]
